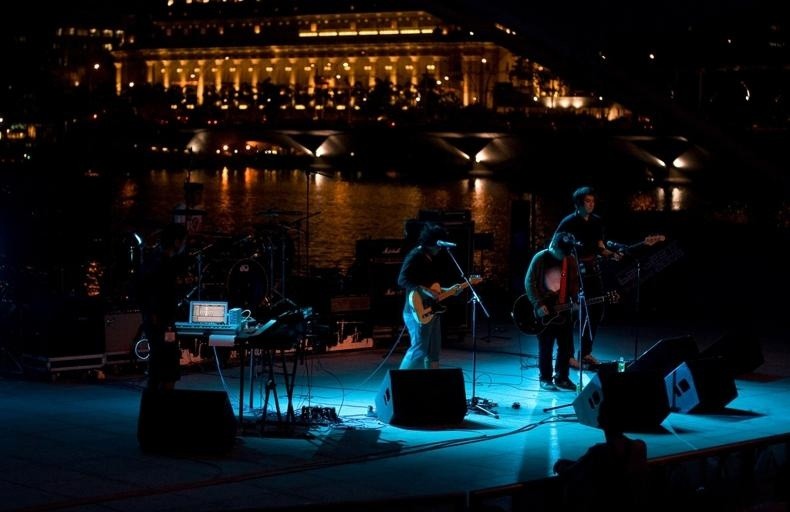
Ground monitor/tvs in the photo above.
[190,300,228,325]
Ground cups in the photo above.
[228,308,241,326]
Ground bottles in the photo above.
[618,357,625,373]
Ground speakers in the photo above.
[375,368,467,427]
[624,335,738,415]
[573,359,672,430]
[136,387,240,465]
[695,323,767,375]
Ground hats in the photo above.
[553,231,584,248]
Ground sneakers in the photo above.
[539,379,557,390]
[570,350,603,371]
[553,374,576,389]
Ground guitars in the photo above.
[409,275,484,324]
[513,290,621,335]
[576,234,666,284]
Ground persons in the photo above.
[554,185,626,372]
[135,228,189,456]
[181,179,205,209]
[524,231,578,393]
[395,225,463,372]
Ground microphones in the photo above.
[607,240,627,248]
[563,235,581,246]
[436,240,458,247]
[189,242,213,257]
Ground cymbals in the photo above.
[252,222,291,237]
[190,243,213,257]
[254,210,287,217]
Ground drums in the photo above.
[199,258,268,313]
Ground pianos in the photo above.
[175,322,241,336]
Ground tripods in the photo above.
[447,248,498,417]
[543,245,594,413]
